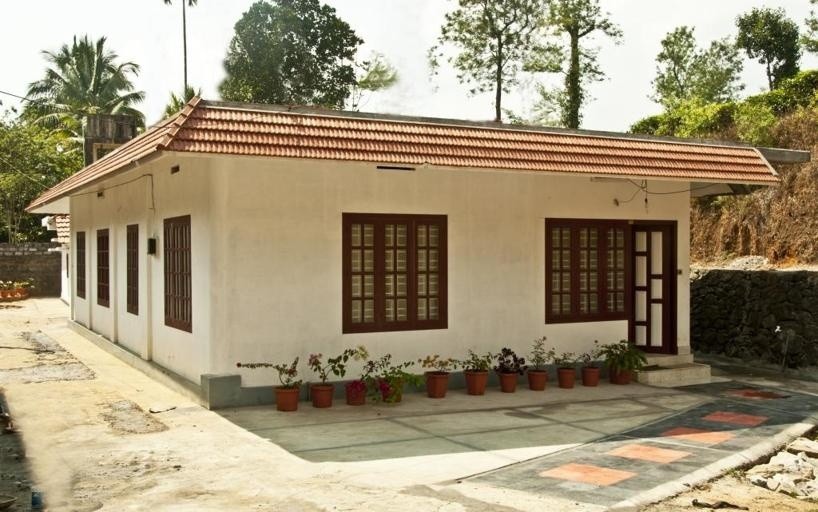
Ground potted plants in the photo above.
[236,354,304,414]
